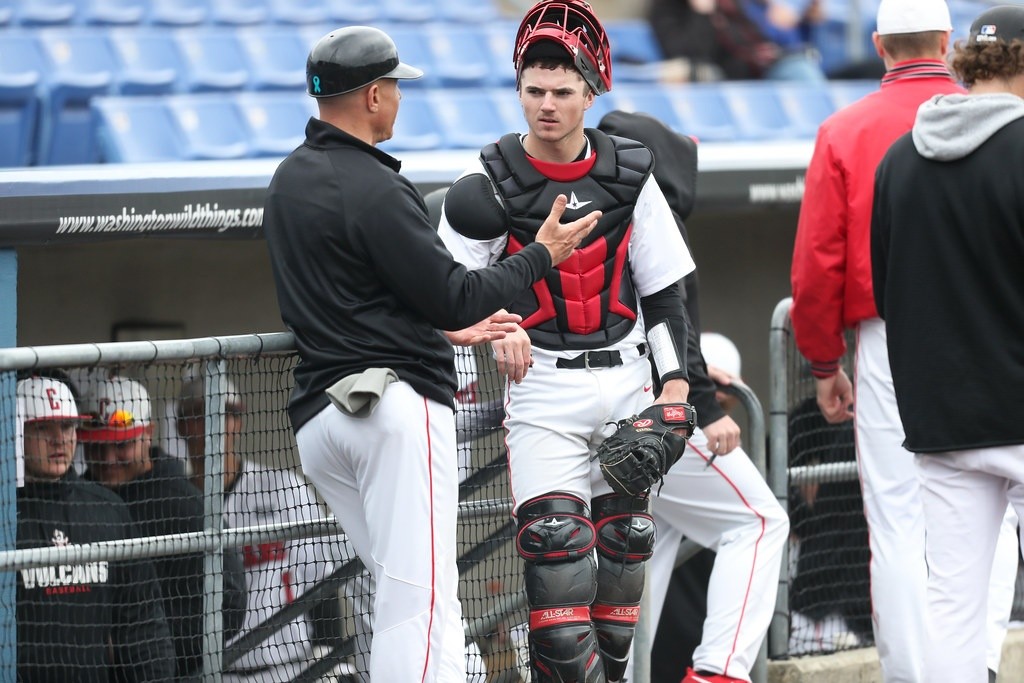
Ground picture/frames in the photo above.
[109,323,187,342]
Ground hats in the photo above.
[877,0,953,35]
[964,5,1024,48]
[16,377,92,424]
[73,377,151,443]
[176,368,242,417]
[699,333,741,379]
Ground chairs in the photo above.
[0,0,988,167]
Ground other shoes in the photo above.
[680,668,748,683]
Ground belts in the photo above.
[528,343,645,369]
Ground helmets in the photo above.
[306,26,423,97]
[513,0,612,96]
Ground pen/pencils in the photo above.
[704,449,718,471]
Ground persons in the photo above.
[78,376,248,682]
[262,25,604,683]
[318,508,378,683]
[646,0,889,84]
[428,0,696,683]
[179,372,346,683]
[793,0,973,682]
[18,374,181,683]
[652,331,885,683]
[869,5,1024,682]
[594,110,792,683]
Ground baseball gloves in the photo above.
[588,402,699,503]
